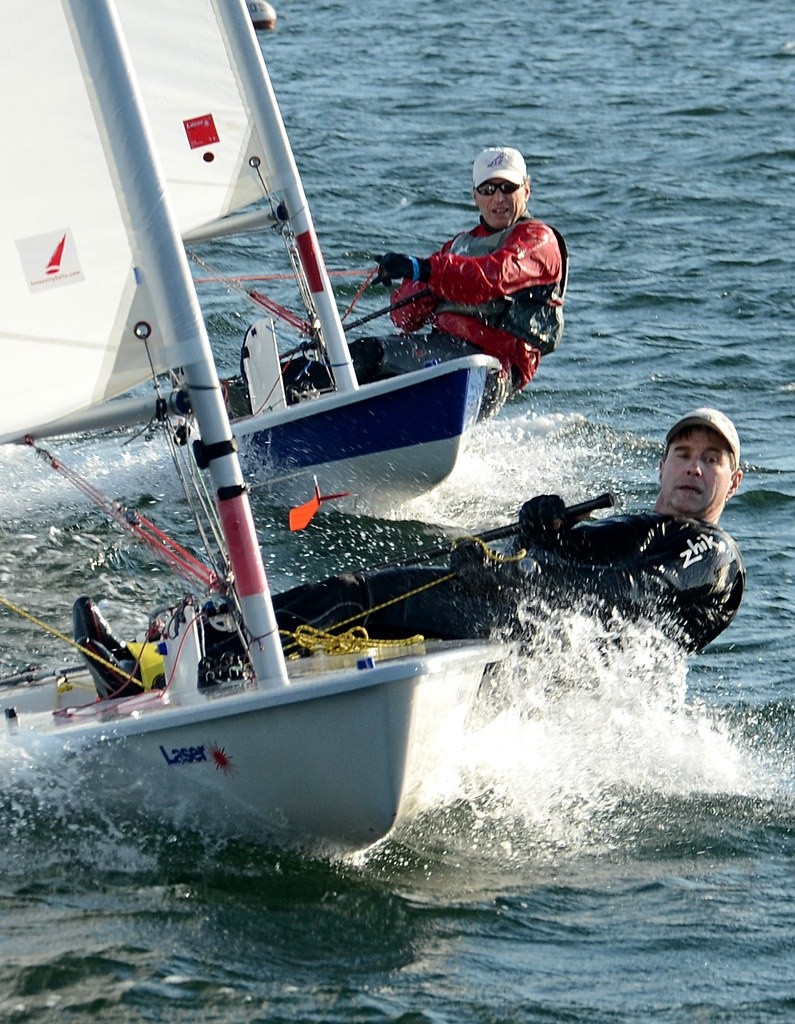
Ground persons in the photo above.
[296,146,568,425]
[72,409,745,697]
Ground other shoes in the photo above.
[72,594,162,700]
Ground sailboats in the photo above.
[106,1,503,515]
[0,0,517,878]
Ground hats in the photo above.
[472,145,528,190]
[664,407,740,472]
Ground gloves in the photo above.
[519,494,568,546]
[371,251,431,287]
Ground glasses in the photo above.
[475,181,524,196]
